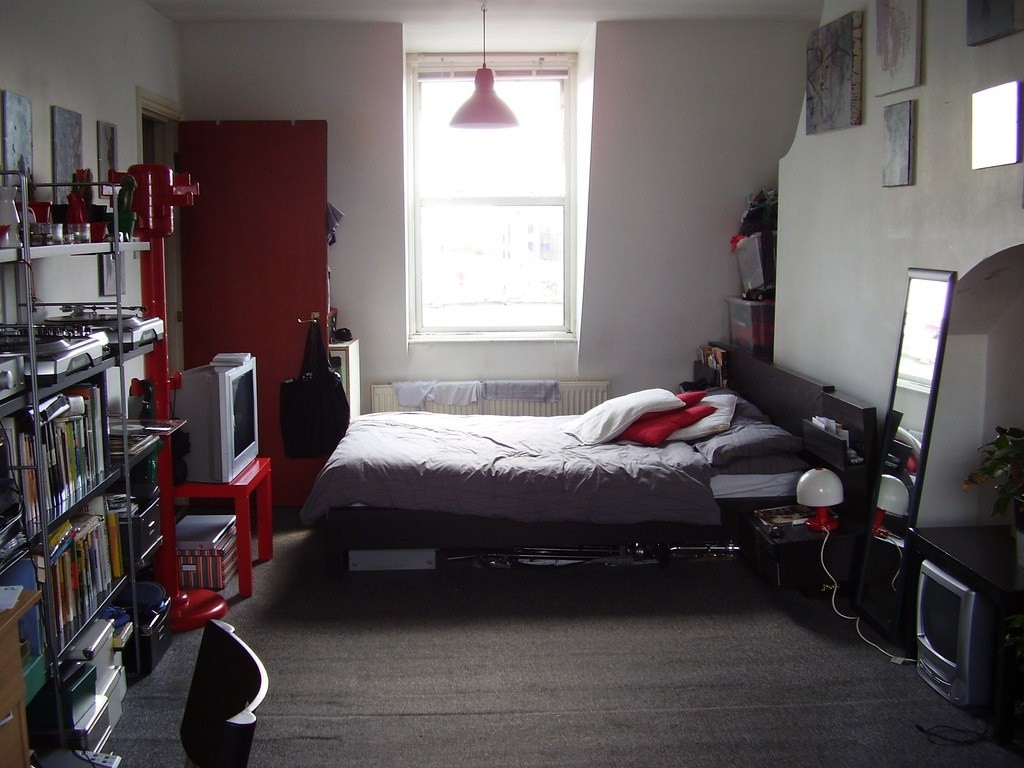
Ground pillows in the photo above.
[562,389,739,445]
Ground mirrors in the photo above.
[866,266,958,636]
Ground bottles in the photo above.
[66,190,87,224]
[0,186,24,248]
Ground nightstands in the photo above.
[873,533,905,619]
[749,509,846,603]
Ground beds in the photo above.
[299,343,876,593]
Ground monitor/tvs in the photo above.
[128,355,259,484]
[916,559,997,711]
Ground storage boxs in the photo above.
[176,515,237,590]
[128,597,174,673]
[725,295,775,362]
[122,499,162,559]
[734,229,777,297]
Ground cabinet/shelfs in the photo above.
[0,170,162,768]
[904,524,1024,756]
[329,339,361,422]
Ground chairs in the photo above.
[180,617,270,768]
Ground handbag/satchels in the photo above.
[278,318,350,460]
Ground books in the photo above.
[0,383,111,521]
[110,432,160,455]
[0,496,124,655]
[105,493,139,517]
[697,344,728,387]
[210,352,251,366]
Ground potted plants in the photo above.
[961,425,1024,568]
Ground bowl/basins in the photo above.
[16,201,52,224]
[90,221,112,243]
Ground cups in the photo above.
[19,222,91,246]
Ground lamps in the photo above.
[449,1,521,128]
[872,475,908,537]
[796,466,844,531]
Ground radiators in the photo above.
[372,381,612,417]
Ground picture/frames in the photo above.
[97,121,118,198]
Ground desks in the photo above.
[171,457,273,597]
